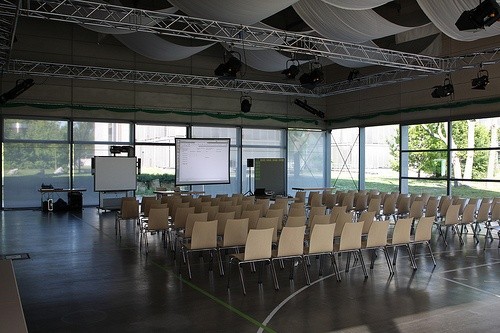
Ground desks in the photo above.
[152,190,205,199]
[292,187,336,192]
[37,188,86,210]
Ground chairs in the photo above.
[114,189,500,296]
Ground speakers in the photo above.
[247,159,253,167]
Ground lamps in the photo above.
[240,93,252,112]
[299,55,324,90]
[215,44,242,80]
[431,73,455,98]
[455,0,500,33]
[347,69,365,82]
[472,65,489,90]
[281,53,301,78]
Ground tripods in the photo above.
[243,167,253,196]
